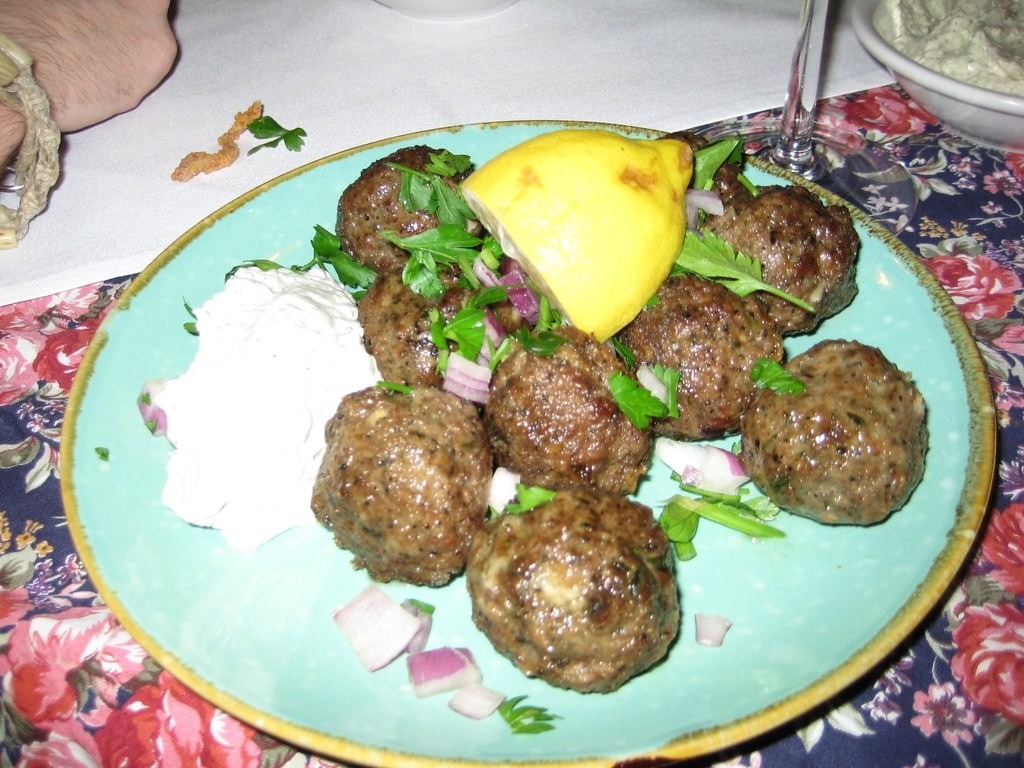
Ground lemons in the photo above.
[460,128,695,347]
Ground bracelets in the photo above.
[0,34,64,251]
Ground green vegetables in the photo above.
[288,131,818,554]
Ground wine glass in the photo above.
[693,0,919,237]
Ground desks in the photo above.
[0,0,1024,768]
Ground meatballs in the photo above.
[308,144,930,693]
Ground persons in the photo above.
[0,0,179,177]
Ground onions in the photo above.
[135,375,171,437]
[332,586,507,720]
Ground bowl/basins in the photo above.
[851,0,1024,155]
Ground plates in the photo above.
[61,120,996,768]
